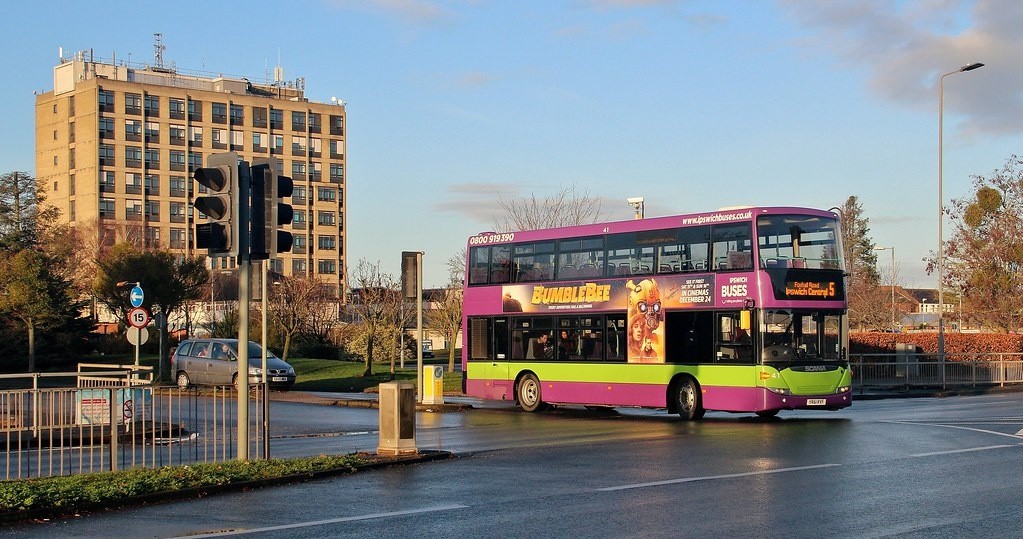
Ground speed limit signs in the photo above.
[128,307,149,329]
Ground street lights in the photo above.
[875,245,895,339]
[922,298,927,331]
[937,62,985,388]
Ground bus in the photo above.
[462,204,853,422]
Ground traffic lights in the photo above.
[193,150,240,257]
[252,156,294,257]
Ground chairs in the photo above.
[472,255,807,285]
[526,336,613,359]
[731,327,745,357]
[214,347,222,358]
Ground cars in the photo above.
[422,347,435,359]
[170,337,296,393]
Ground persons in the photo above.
[197,345,208,356]
[561,330,577,353]
[533,332,553,357]
[216,344,228,357]
[629,314,647,357]
[643,336,657,357]
